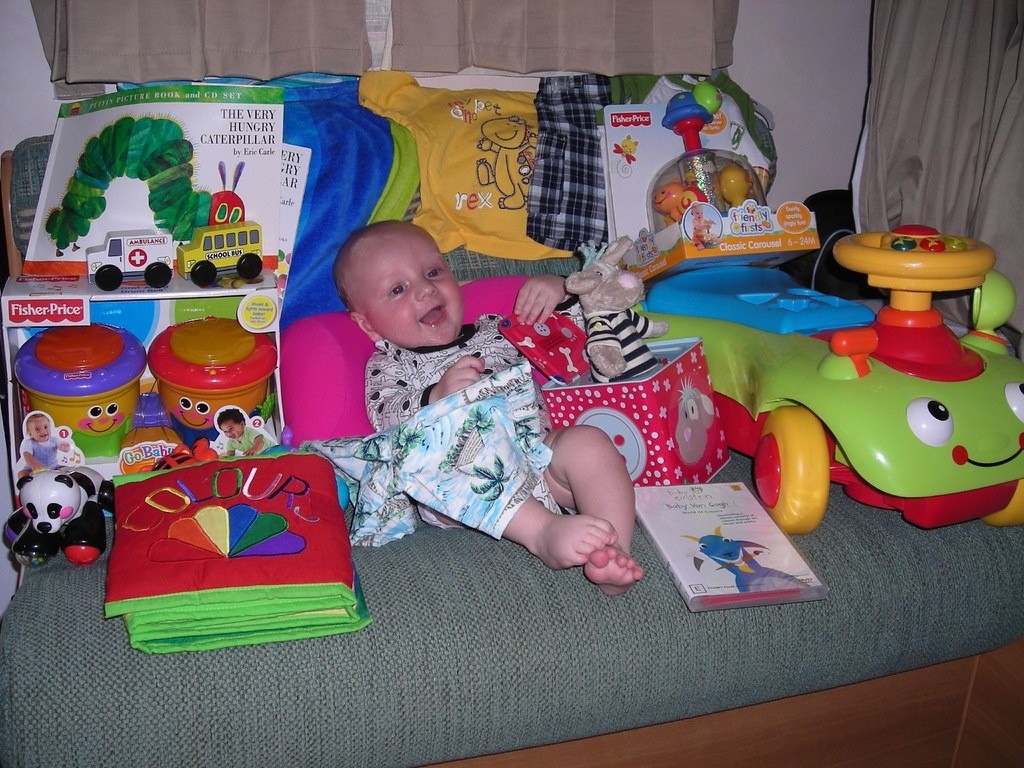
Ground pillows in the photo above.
[280,275,550,448]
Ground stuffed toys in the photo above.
[567,237,667,379]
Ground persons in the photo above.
[17,414,75,480]
[337,216,643,596]
[217,409,275,457]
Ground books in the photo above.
[632,482,830,616]
[24,83,314,328]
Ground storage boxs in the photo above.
[596,82,821,283]
[1,271,284,518]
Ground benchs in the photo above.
[0,129,1024,768]
